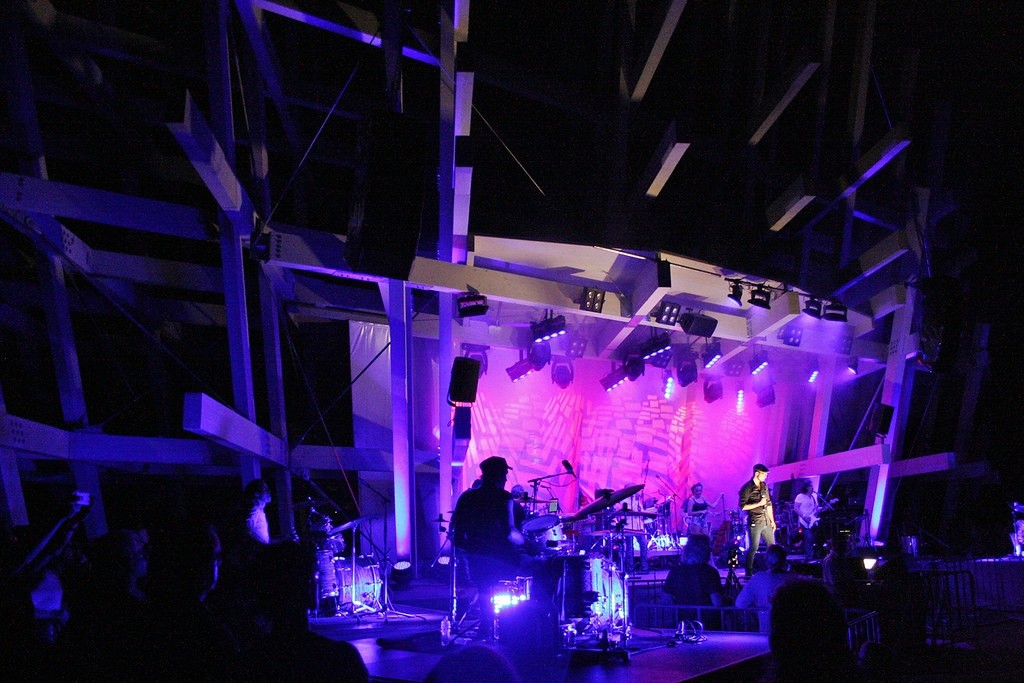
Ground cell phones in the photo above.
[73,491,90,505]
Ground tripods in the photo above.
[316,502,415,628]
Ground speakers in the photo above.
[917,304,976,396]
[680,313,718,338]
[450,357,480,402]
[344,111,429,282]
[869,402,894,435]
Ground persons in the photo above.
[448,456,616,618]
[760,583,894,683]
[623,483,649,574]
[794,480,836,559]
[662,534,724,631]
[735,544,813,634]
[824,535,850,591]
[738,464,776,577]
[688,482,725,569]
[0,480,369,683]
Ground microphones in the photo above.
[562,459,573,471]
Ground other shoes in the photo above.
[744,572,754,577]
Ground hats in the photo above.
[754,463,770,473]
[480,457,515,473]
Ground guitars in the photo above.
[799,497,840,530]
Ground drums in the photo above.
[314,549,339,600]
[311,518,346,558]
[521,515,564,552]
[539,553,629,629]
[559,512,577,546]
[334,557,381,608]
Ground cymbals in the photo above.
[574,483,646,521]
[331,517,367,535]
[592,510,658,519]
[584,528,650,536]
[513,496,555,504]
